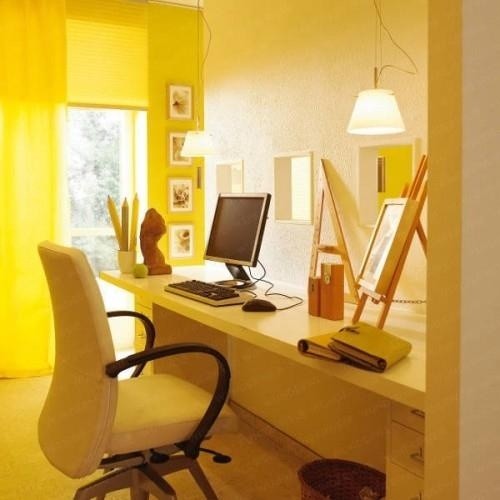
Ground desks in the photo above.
[101,266,426,500]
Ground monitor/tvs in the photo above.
[202,191,272,291]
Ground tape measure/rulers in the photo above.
[310,157,361,304]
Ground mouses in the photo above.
[241,298,277,313]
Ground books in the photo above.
[298,330,346,363]
[330,320,415,374]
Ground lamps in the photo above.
[347,0,418,135]
[180,0,220,158]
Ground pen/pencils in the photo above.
[105,192,139,249]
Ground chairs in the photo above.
[38,242,232,499]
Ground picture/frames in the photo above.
[357,198,417,295]
[167,222,194,258]
[167,176,192,210]
[167,83,194,120]
[168,131,191,166]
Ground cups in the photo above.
[116,249,137,273]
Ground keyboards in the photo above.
[164,279,246,308]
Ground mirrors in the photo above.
[274,152,314,225]
[358,142,414,228]
[216,161,243,194]
